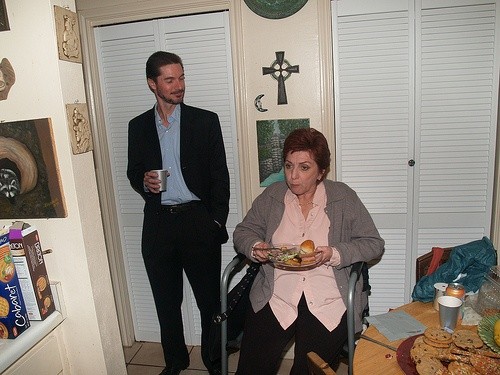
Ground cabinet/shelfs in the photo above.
[1,325,73,375]
[330,0,500,316]
[94,10,243,347]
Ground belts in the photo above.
[145,199,203,217]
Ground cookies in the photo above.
[410,319,500,375]
[37,278,51,310]
[0,297,9,316]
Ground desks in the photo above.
[353,301,476,375]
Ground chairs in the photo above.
[219,253,370,375]
[416,247,497,283]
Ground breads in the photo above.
[284,239,316,265]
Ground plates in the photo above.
[396,334,452,375]
[477,311,500,354]
[266,243,322,271]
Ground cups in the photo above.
[433,282,449,311]
[437,296,462,330]
[150,170,168,192]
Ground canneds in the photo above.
[446,284,465,317]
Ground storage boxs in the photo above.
[0,224,31,339]
[8,219,55,321]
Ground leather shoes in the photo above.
[207,367,221,375]
[159,360,190,375]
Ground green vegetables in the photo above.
[269,251,298,260]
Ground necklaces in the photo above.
[299,200,313,206]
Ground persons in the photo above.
[233,127,385,375]
[127,51,230,375]
